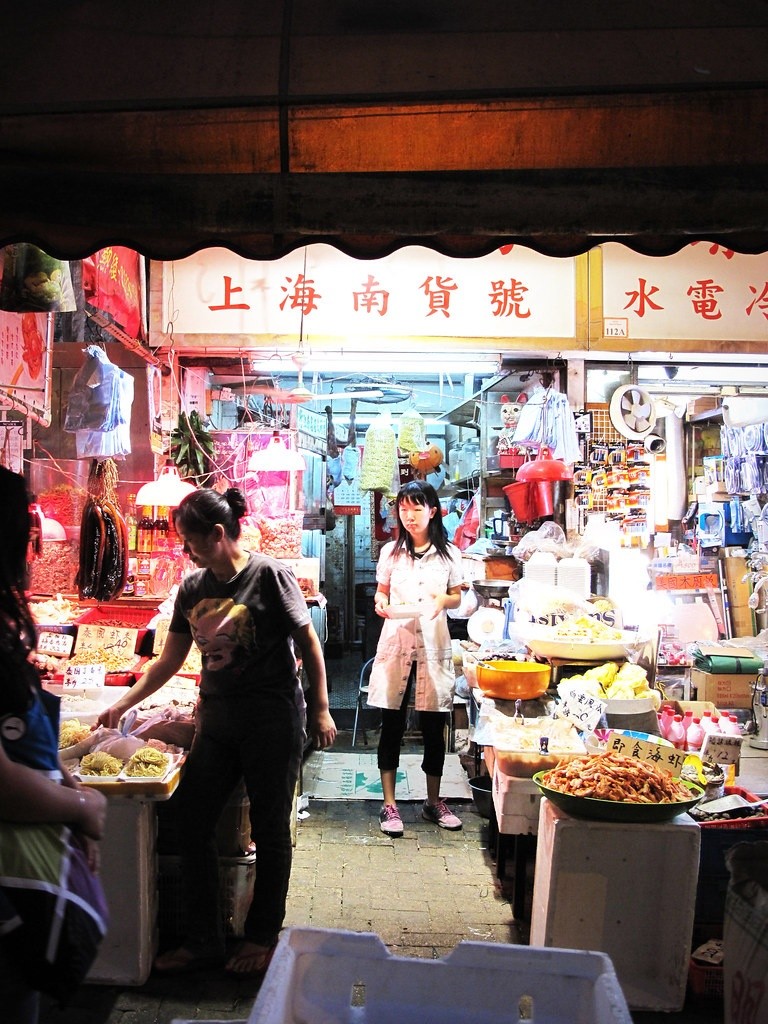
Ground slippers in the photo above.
[223,941,276,978]
[151,945,201,977]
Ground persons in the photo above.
[366,480,462,833]
[90,488,338,972]
[0,464,107,1024]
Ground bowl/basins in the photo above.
[469,777,492,817]
[533,769,705,819]
[476,661,551,700]
[523,552,591,600]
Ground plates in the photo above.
[522,631,649,658]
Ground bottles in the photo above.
[657,707,741,753]
[125,501,168,553]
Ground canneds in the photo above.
[122,553,152,598]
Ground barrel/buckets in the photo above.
[503,480,552,522]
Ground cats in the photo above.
[496,393,531,452]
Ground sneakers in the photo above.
[378,804,404,833]
[422,797,462,828]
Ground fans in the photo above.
[239,351,385,403]
[344,377,415,404]
[610,384,659,439]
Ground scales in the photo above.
[445,558,478,622]
[467,577,517,648]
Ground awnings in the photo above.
[0,161,768,261]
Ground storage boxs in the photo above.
[490,758,540,837]
[76,799,156,987]
[723,555,756,636]
[701,829,768,878]
[688,924,723,997]
[74,605,157,654]
[159,852,258,939]
[698,496,707,504]
[129,655,202,685]
[53,655,145,686]
[486,454,540,470]
[154,793,251,854]
[689,458,703,466]
[695,877,728,922]
[483,743,495,779]
[705,481,726,494]
[30,620,74,646]
[706,492,743,502]
[690,668,768,708]
[688,494,697,501]
[695,476,705,495]
[244,923,636,1024]
[695,784,768,829]
[689,466,704,476]
[659,699,743,778]
[529,794,703,1015]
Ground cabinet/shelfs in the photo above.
[448,367,555,547]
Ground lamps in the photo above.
[247,349,506,376]
[248,381,306,473]
[134,355,198,508]
[33,441,69,542]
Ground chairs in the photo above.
[350,655,407,748]
